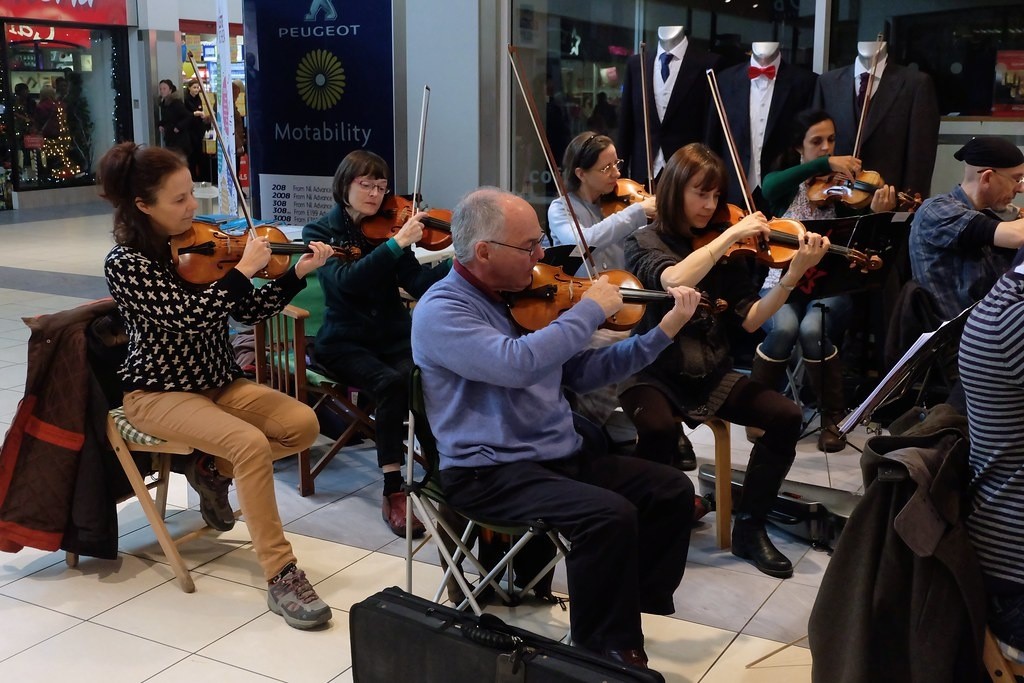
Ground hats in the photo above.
[954,137,1024,169]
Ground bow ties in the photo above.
[748,65,775,79]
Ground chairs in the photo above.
[0,263,1024,683]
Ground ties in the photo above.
[660,53,673,82]
[857,73,870,116]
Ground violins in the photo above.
[807,170,922,213]
[507,262,728,331]
[693,203,883,274]
[600,179,656,219]
[170,220,362,285]
[361,196,454,252]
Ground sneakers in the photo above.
[267,564,333,630]
[185,454,236,532]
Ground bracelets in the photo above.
[705,245,718,266]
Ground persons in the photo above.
[549,91,617,145]
[11,65,83,177]
[535,109,1024,578]
[410,186,701,670]
[302,152,457,539]
[94,141,333,630]
[704,41,823,211]
[807,41,941,198]
[155,78,248,201]
[607,25,724,194]
[955,260,1024,683]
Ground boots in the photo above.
[747,344,795,443]
[803,345,847,452]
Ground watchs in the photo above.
[776,281,796,293]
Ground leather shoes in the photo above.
[677,434,698,469]
[732,517,793,577]
[609,647,650,668]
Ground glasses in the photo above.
[489,230,547,256]
[597,158,624,175]
[355,180,391,194]
[977,168,1024,184]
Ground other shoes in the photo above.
[382,490,426,537]
